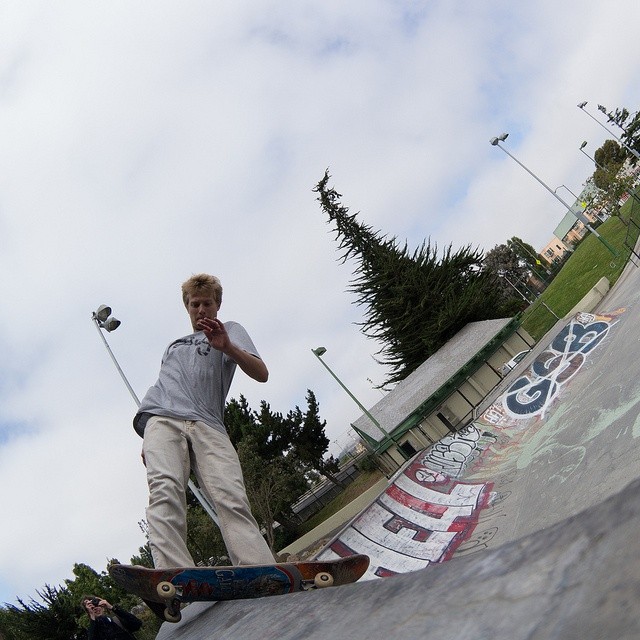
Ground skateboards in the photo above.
[108,552,371,623]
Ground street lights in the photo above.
[310,346,413,462]
[504,270,531,304]
[510,269,539,300]
[554,184,605,223]
[488,131,619,259]
[576,101,640,162]
[578,140,640,201]
[90,304,226,536]
[508,240,555,279]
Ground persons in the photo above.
[133,273,278,570]
[79,594,142,639]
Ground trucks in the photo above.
[496,350,532,378]
